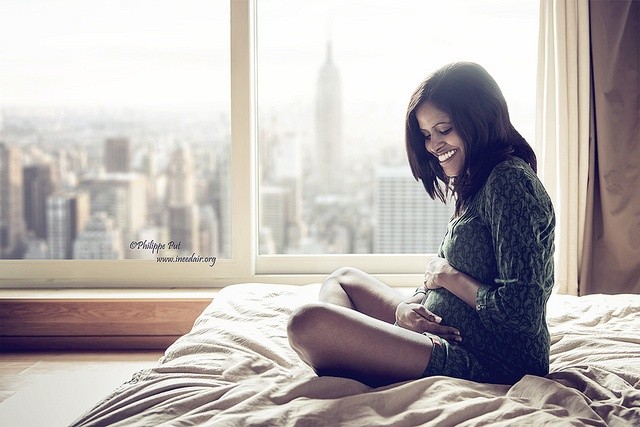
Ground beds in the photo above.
[70,283,640,426]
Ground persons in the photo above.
[285,58,556,388]
